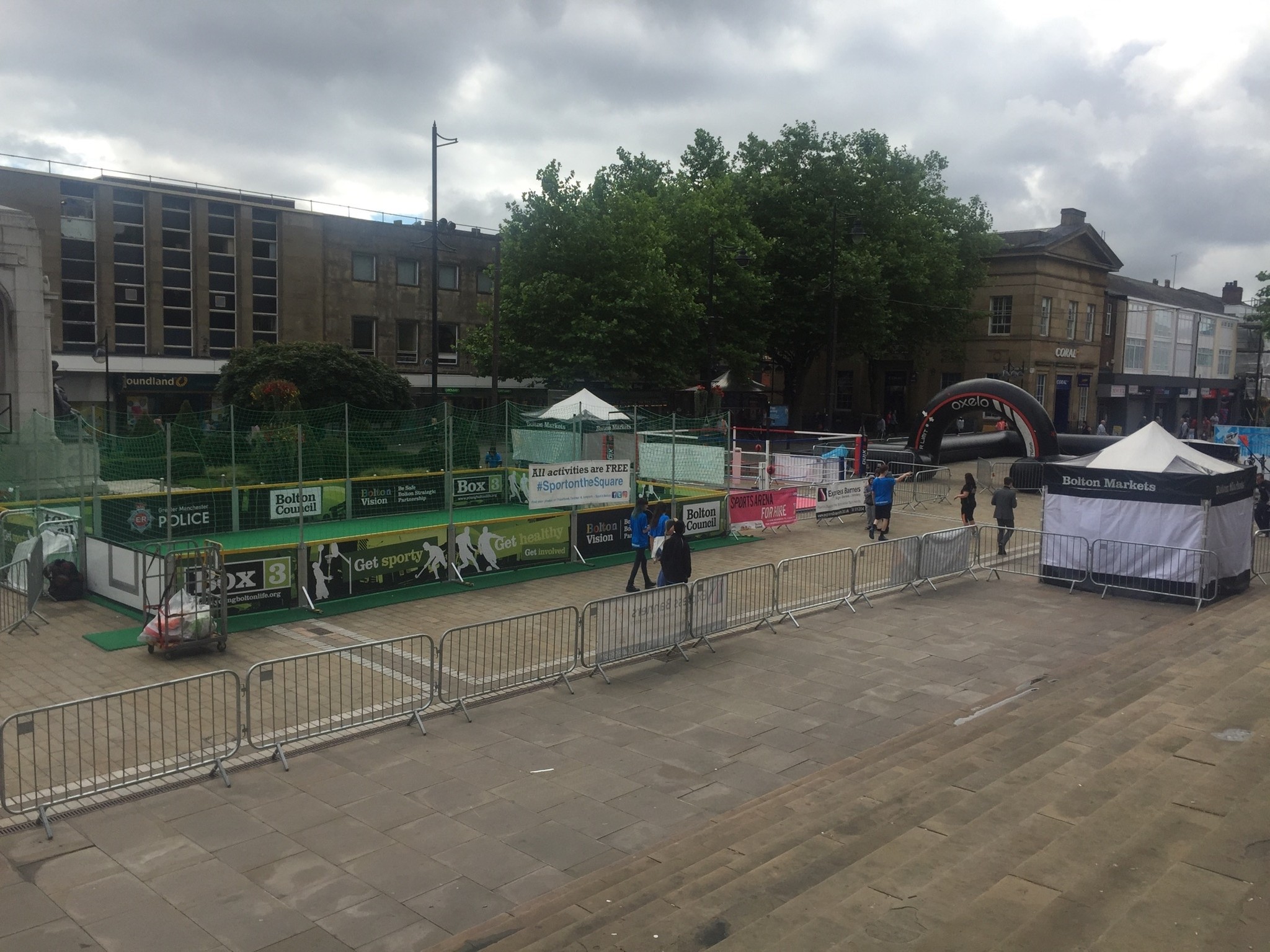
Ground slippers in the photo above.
[644,577,656,589]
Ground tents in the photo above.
[519,388,630,422]
[1041,421,1258,607]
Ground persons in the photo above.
[625,498,657,592]
[995,417,1005,431]
[991,477,1017,553]
[954,472,976,534]
[485,446,503,468]
[1081,408,1229,441]
[863,465,913,541]
[716,408,900,442]
[657,518,691,589]
[649,501,670,564]
[1247,453,1270,537]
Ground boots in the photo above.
[626,580,640,592]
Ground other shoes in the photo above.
[998,543,1006,555]
[878,535,888,541]
[869,528,874,539]
